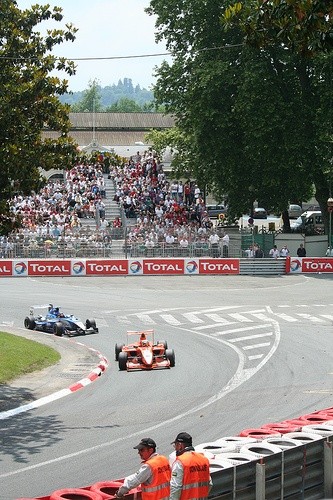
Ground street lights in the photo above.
[327,197,333,251]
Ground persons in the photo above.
[244,246,255,258]
[168,432,213,500]
[325,245,333,257]
[140,339,149,348]
[253,242,258,252]
[298,221,325,236]
[279,245,289,257]
[296,243,306,257]
[255,246,264,258]
[48,308,65,318]
[0,147,229,259]
[112,438,171,500]
[268,245,279,258]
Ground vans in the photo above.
[206,205,227,217]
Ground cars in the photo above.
[288,204,302,218]
[291,210,324,226]
[253,208,267,219]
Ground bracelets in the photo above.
[115,490,123,497]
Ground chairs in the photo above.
[50,309,55,314]
[140,337,146,347]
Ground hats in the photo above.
[134,437,156,450]
[171,432,192,444]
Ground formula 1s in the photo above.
[115,329,174,370]
[24,304,98,338]
